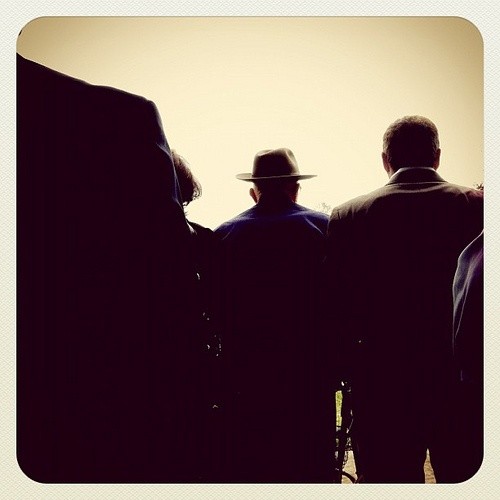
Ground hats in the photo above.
[235,148,317,180]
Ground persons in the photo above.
[198,148,337,481]
[321,111,482,482]
